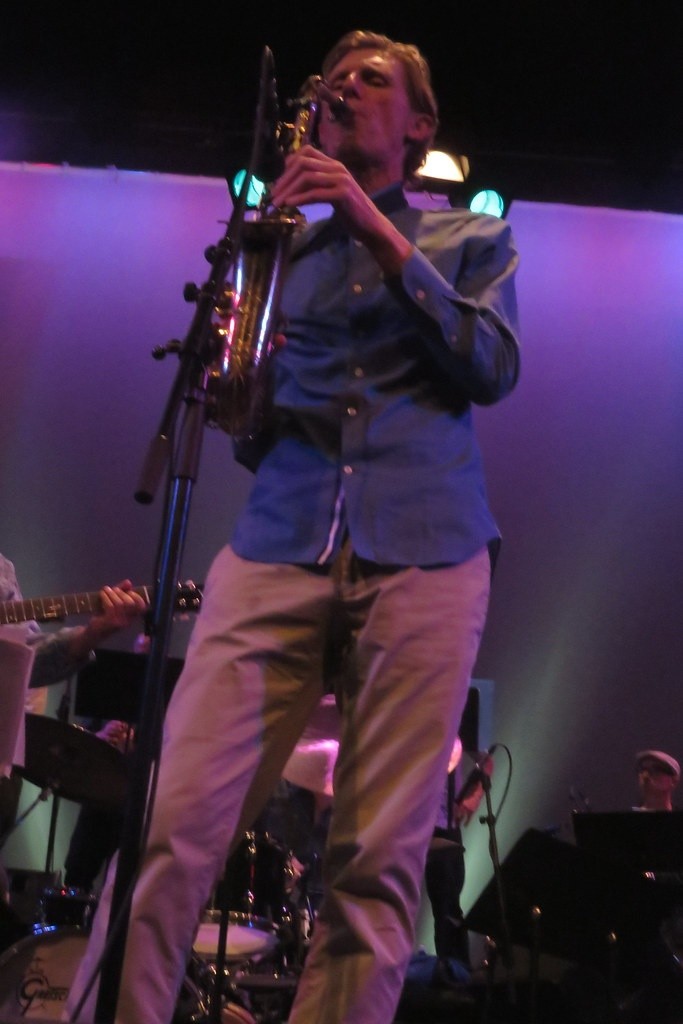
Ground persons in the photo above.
[0,553,683,1023]
[57,29,523,1023]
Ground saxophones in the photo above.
[150,74,355,439]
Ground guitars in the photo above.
[0,579,204,626]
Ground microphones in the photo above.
[453,747,496,806]
[251,45,287,182]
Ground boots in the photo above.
[424,846,473,985]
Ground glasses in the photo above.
[633,765,674,776]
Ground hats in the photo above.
[632,750,680,776]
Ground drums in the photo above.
[192,829,287,963]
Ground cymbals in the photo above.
[22,713,142,813]
